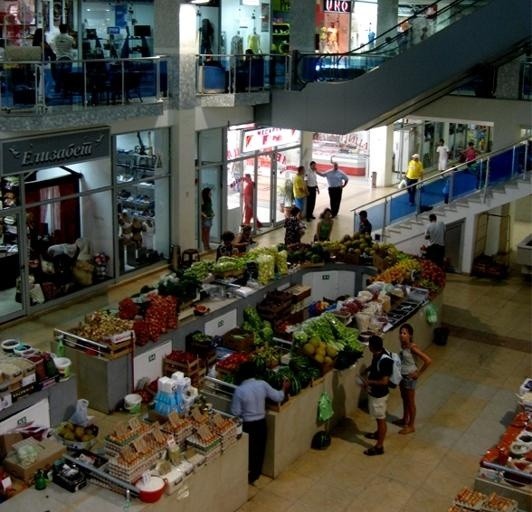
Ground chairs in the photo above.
[7,53,322,106]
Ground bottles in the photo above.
[35,468,46,490]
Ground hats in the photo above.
[411,153,420,159]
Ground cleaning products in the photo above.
[54,333,65,355]
[155,372,198,419]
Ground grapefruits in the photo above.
[302,336,337,367]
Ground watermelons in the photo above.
[224,356,321,394]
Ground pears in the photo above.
[58,422,97,441]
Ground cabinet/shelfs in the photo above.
[114,149,157,228]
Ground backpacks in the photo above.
[377,348,402,385]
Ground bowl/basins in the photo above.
[136,477,166,503]
[58,424,100,451]
[360,330,376,342]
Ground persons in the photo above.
[231,31,244,61]
[319,22,339,53]
[230,359,291,484]
[31,22,85,103]
[426,215,447,268]
[203,51,221,67]
[368,30,375,49]
[436,139,450,176]
[247,30,260,55]
[199,19,214,64]
[384,26,429,57]
[392,324,433,434]
[201,162,371,261]
[461,141,481,168]
[117,212,146,246]
[2,4,21,46]
[240,49,255,66]
[405,154,423,206]
[359,336,393,455]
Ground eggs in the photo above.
[448,485,510,512]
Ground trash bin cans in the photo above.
[433,328,449,346]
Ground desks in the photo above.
[0,234,445,512]
[448,377,531,512]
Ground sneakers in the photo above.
[363,432,383,440]
[362,446,385,456]
[301,216,316,222]
[393,420,415,434]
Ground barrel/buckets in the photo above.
[1,338,47,382]
[123,393,143,413]
[55,357,72,381]
